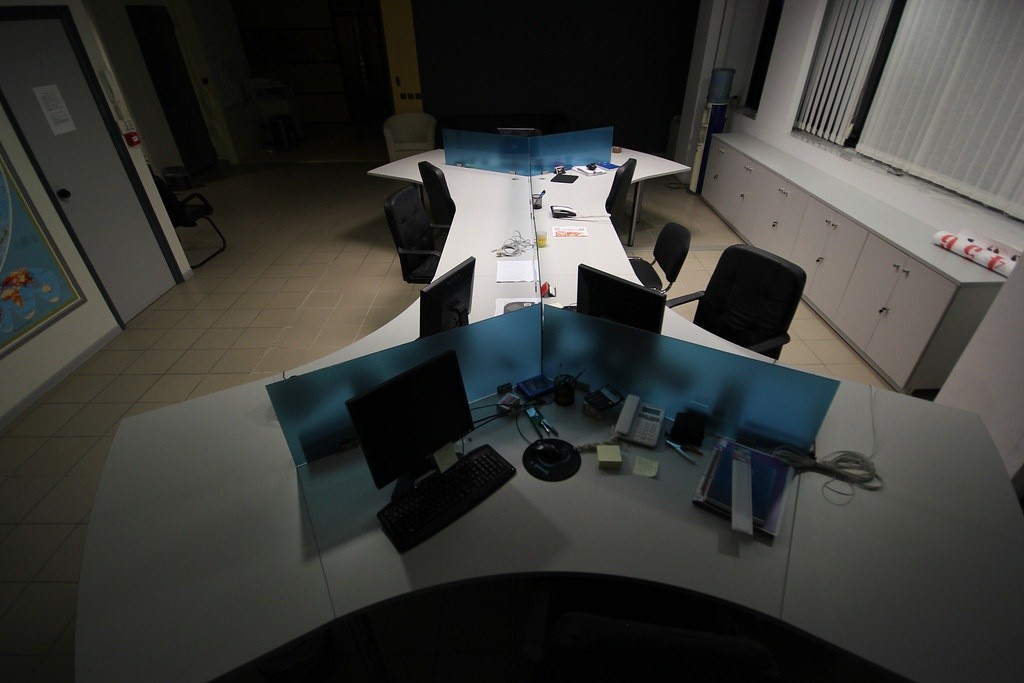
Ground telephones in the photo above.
[614,393,665,447]
[550,205,576,218]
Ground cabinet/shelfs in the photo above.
[326,0,397,127]
[700,132,1012,401]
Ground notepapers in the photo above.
[597,445,622,469]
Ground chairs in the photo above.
[604,157,808,361]
[383,112,437,163]
[384,161,456,285]
[496,127,543,153]
[521,577,786,683]
[149,166,226,270]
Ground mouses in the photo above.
[532,439,559,462]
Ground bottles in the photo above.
[707,68,736,103]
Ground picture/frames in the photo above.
[0,140,88,362]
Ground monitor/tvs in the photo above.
[577,264,667,336]
[419,256,477,338]
[345,348,475,492]
[498,128,540,156]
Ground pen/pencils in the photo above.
[569,370,585,386]
[535,189,546,203]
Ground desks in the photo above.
[71,126,1024,683]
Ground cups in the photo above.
[553,374,576,406]
[532,194,542,209]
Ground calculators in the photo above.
[584,384,624,412]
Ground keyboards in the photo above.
[375,444,516,555]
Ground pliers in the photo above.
[665,439,704,464]
